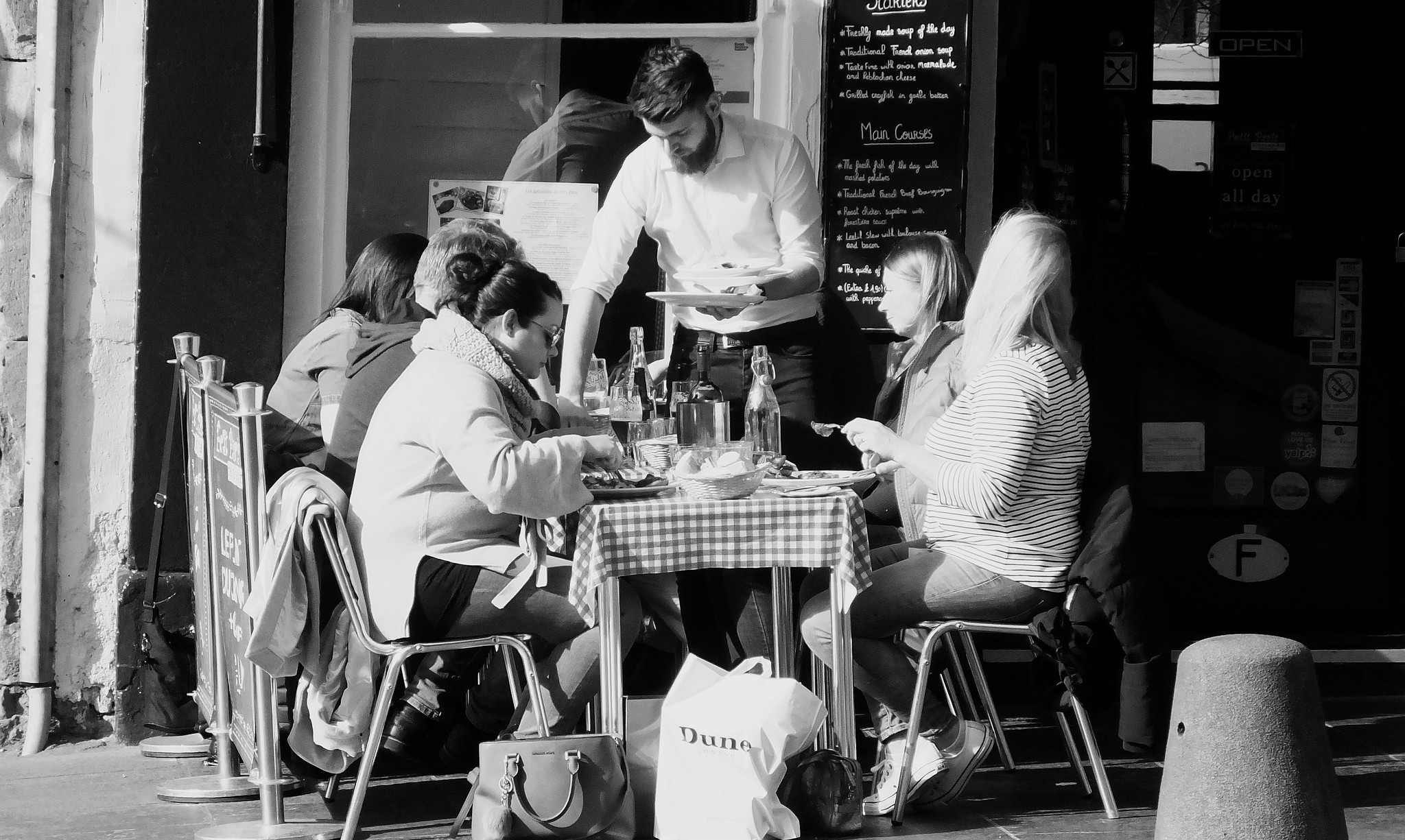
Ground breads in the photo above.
[611,473,669,487]
[811,421,833,437]
[798,471,839,479]
[764,455,794,479]
[676,451,756,477]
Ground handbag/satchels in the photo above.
[450,734,635,839]
[780,748,863,836]
[141,603,199,733]
[653,651,830,840]
[623,695,665,837]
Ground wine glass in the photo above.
[608,385,643,465]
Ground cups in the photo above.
[629,417,677,467]
[582,358,608,411]
[669,440,754,468]
[670,379,694,417]
[676,400,731,445]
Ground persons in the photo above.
[799,213,1093,815]
[491,47,648,214]
[555,48,827,677]
[715,233,976,679]
[259,219,645,783]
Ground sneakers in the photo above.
[862,734,948,817]
[919,720,994,809]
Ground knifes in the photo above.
[614,470,627,482]
[783,481,854,493]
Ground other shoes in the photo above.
[380,702,491,773]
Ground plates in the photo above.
[761,470,876,487]
[588,479,682,496]
[645,291,767,309]
[771,485,841,497]
[673,256,794,287]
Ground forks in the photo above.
[824,423,843,430]
[606,471,618,479]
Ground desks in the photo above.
[519,440,873,763]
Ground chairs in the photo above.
[265,285,1158,840]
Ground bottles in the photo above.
[583,352,600,413]
[745,344,782,465]
[622,326,657,458]
[687,344,724,403]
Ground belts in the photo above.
[680,312,819,349]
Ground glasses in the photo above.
[527,319,565,348]
